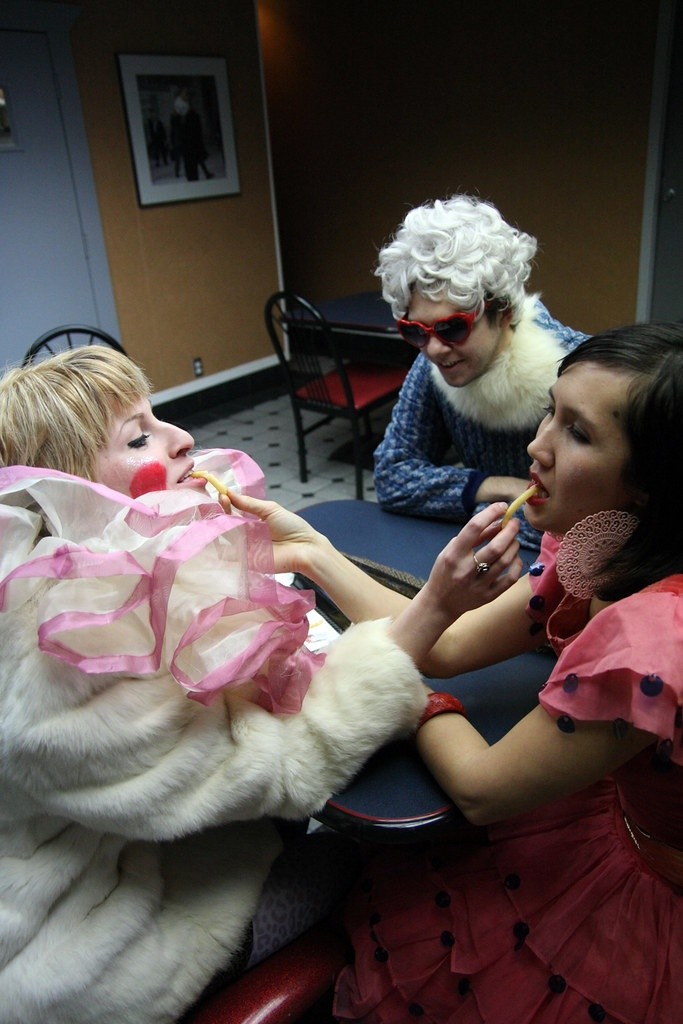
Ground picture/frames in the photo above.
[0,81,25,155]
[116,51,242,209]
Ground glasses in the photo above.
[397,300,491,348]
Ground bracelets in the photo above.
[409,692,468,738]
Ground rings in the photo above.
[473,555,490,575]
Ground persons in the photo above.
[1,347,523,1024]
[217,324,683,1024]
[372,196,586,514]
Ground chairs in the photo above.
[264,291,412,500]
[22,323,127,367]
[179,931,345,1024]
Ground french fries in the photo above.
[189,470,227,494]
[502,484,538,530]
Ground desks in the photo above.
[278,289,415,367]
[290,500,558,848]
